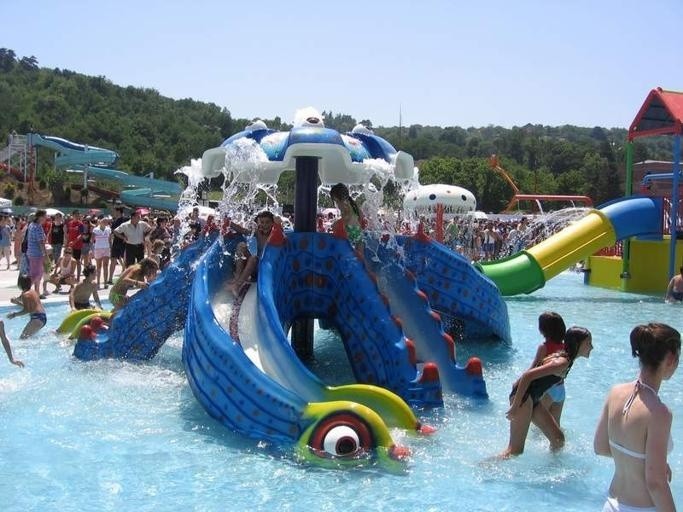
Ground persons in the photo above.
[376,211,566,262]
[481,326,593,460]
[523,311,565,426]
[591,324,681,512]
[665,266,683,304]
[215,183,368,295]
[0,201,215,368]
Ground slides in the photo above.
[328,231,489,420]
[73,228,219,363]
[474,195,659,297]
[395,222,513,346]
[181,215,436,478]
